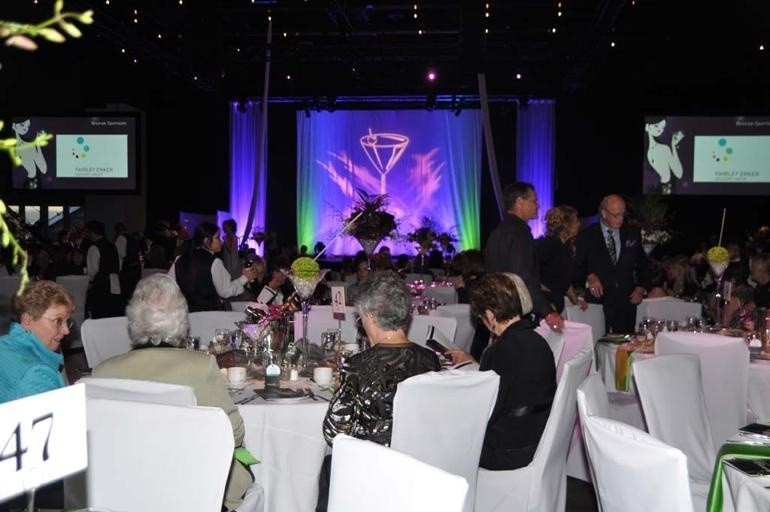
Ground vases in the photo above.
[642,244,657,256]
[709,262,728,293]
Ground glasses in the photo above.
[42,316,73,328]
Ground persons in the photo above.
[642,115,686,198]
[315,275,441,512]
[0,278,78,511]
[444,271,556,472]
[537,206,589,313]
[93,272,259,511]
[1,209,770,349]
[488,181,563,333]
[2,116,49,190]
[577,194,651,341]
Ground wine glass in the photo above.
[186,327,278,368]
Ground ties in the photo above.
[606,230,616,263]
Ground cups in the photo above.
[228,365,247,386]
[314,367,333,387]
[319,328,352,369]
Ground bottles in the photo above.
[409,277,453,315]
[289,364,299,381]
[281,362,289,380]
[626,314,770,353]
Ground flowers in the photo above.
[291,257,320,280]
[707,247,729,265]
[622,179,681,244]
[327,187,463,256]
